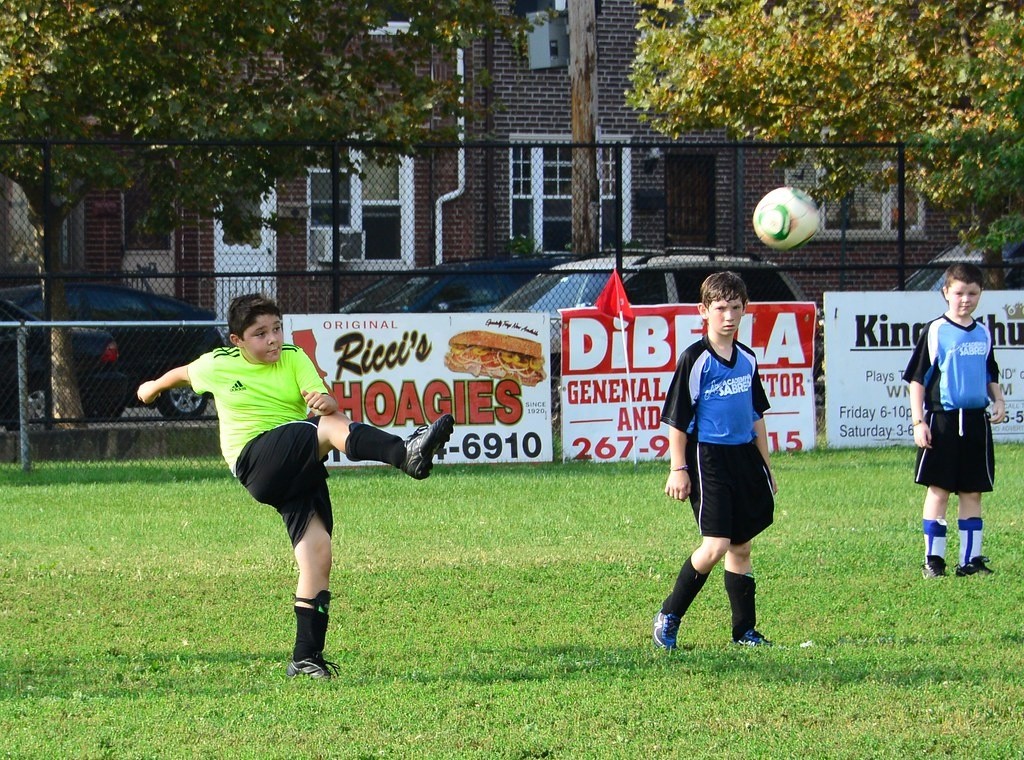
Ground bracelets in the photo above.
[998,399,1005,405]
[670,465,688,472]
[913,419,921,427]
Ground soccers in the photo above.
[751,186,822,253]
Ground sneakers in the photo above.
[400,414,454,480]
[287,653,341,680]
[921,555,946,580]
[954,556,993,577]
[732,629,772,647]
[653,608,681,650]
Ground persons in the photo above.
[652,271,779,650]
[903,261,1007,581]
[136,293,455,681]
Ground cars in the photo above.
[1,282,228,432]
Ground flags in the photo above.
[596,270,633,323]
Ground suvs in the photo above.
[486,246,826,414]
[339,251,587,315]
[892,234,1024,292]
